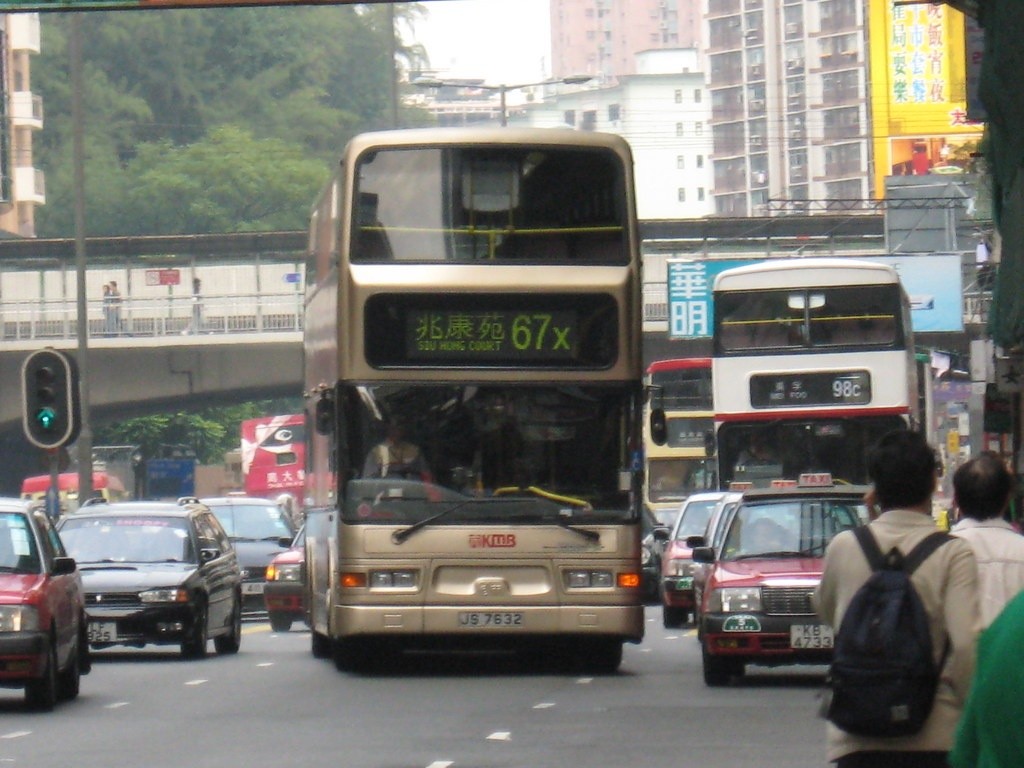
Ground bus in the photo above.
[295,123,669,675]
[240,413,305,515]
[706,254,921,485]
[641,354,715,544]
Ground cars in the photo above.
[200,498,296,613]
[0,497,91,710]
[691,472,881,686]
[651,481,757,630]
[685,476,800,642]
[640,501,669,605]
[263,522,305,632]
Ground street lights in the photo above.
[412,74,592,126]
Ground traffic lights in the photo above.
[22,348,75,448]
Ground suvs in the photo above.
[52,495,244,662]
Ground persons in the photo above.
[342,413,440,499]
[811,429,1024,768]
[181,279,213,335]
[102,281,133,337]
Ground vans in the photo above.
[20,470,130,512]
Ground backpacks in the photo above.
[828,525,957,737]
